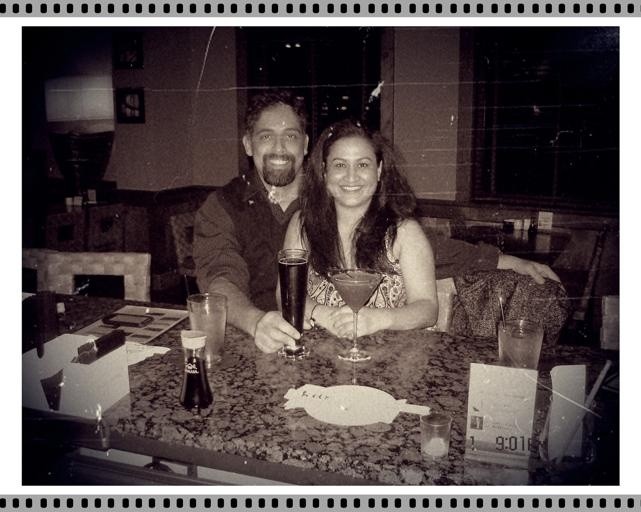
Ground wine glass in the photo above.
[328,269,379,361]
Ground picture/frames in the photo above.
[115,87,144,124]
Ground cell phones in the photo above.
[101,313,153,328]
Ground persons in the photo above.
[188,92,564,357]
[273,119,439,340]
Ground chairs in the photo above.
[22,185,617,349]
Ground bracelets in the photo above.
[309,302,322,326]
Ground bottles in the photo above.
[179,330,214,409]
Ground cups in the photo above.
[277,248,310,358]
[503,218,533,234]
[188,293,227,367]
[496,317,543,370]
[419,412,452,463]
